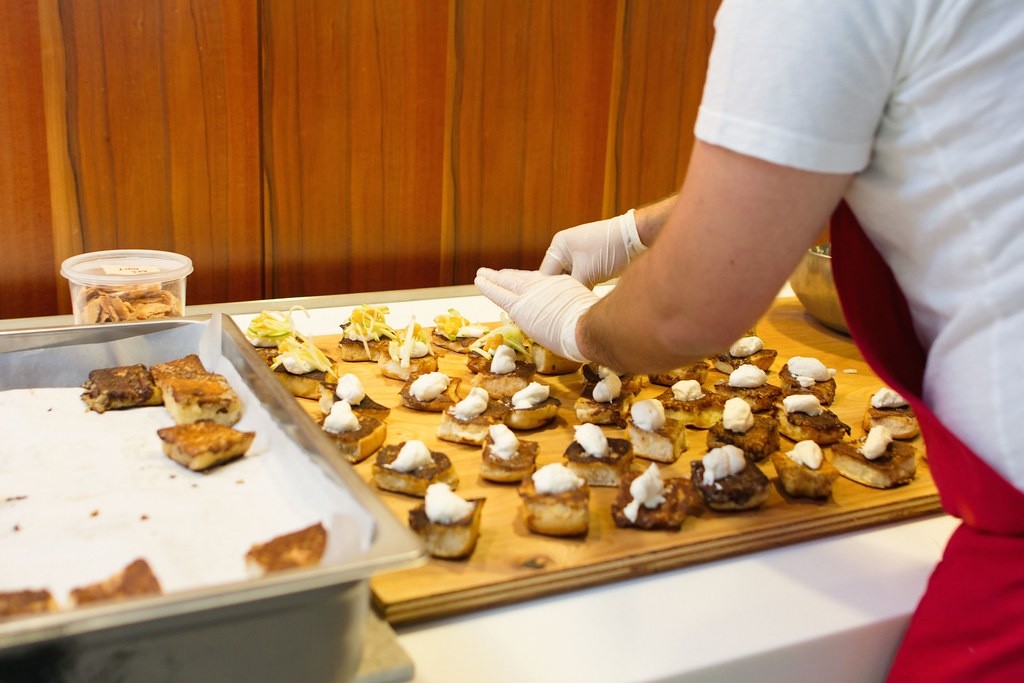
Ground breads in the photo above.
[245,521,328,577]
[0,589,56,623]
[79,351,257,471]
[70,559,162,607]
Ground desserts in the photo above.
[247,305,921,559]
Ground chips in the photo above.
[77,268,176,324]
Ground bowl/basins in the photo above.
[789,248,853,337]
[59,250,194,326]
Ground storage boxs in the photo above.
[0,310,429,682]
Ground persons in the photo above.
[471,0,1022,683]
